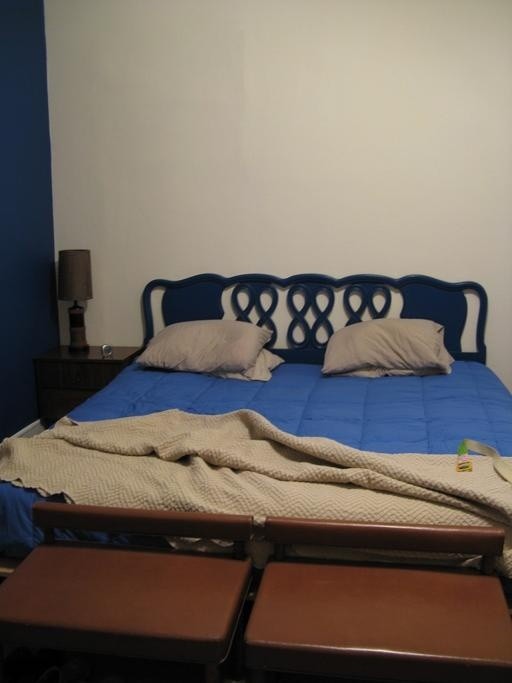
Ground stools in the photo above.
[0,502,512,683]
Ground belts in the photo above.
[456,439,512,481]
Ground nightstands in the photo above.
[33,344,142,427]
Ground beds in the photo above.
[0,273,512,580]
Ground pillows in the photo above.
[213,348,285,381]
[319,317,455,378]
[137,317,274,374]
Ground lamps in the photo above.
[58,248,93,354]
